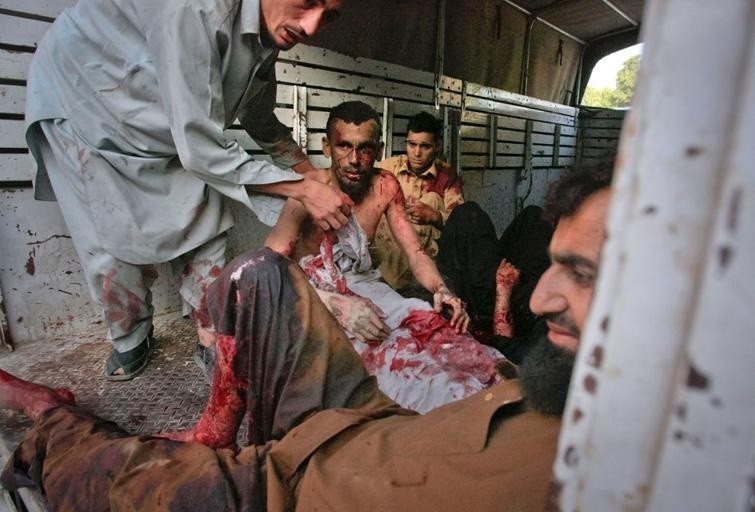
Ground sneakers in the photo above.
[104,325,154,381]
[194,341,215,386]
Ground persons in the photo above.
[265,97,508,415]
[0,146,624,511]
[399,198,563,366]
[369,108,463,293]
[26,0,350,380]
